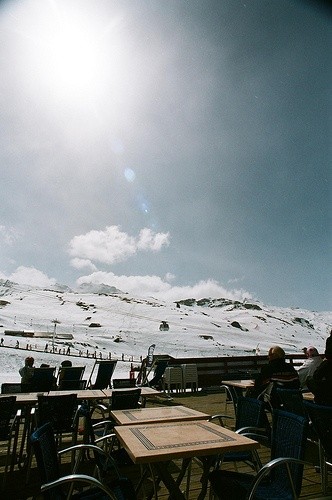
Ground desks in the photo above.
[0,392,48,404]
[110,406,212,425]
[48,390,106,398]
[102,387,161,397]
[114,420,260,500]
[222,379,254,402]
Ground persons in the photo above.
[99,352,102,358]
[16,341,19,347]
[122,353,124,360]
[298,347,323,384]
[45,344,48,351]
[109,352,111,359]
[307,336,332,406]
[60,361,72,367]
[0,338,4,346]
[19,357,35,378]
[66,345,71,355]
[246,346,300,402]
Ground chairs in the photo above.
[0,360,332,500]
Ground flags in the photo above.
[148,344,155,364]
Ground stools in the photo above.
[164,364,198,393]
